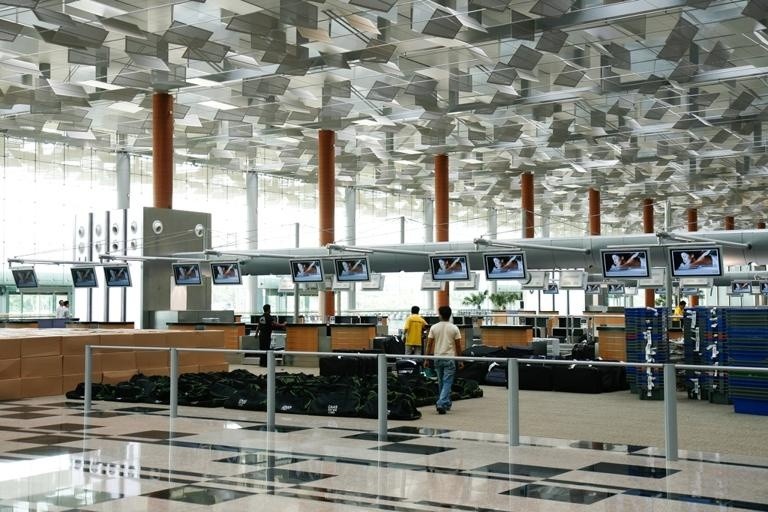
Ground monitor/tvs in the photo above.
[637,266,667,291]
[13,269,38,287]
[71,268,98,287]
[667,244,724,279]
[103,265,132,286]
[522,270,550,291]
[679,276,713,288]
[421,272,446,291]
[482,251,526,280]
[559,270,588,290]
[585,282,601,294]
[257,254,385,296]
[606,281,625,294]
[428,253,471,281]
[172,262,203,286]
[728,264,766,272]
[726,280,768,297]
[599,247,652,280]
[655,287,705,296]
[454,272,480,290]
[209,262,242,285]
[543,282,559,294]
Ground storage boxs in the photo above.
[0,328,229,399]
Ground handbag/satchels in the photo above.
[64,366,486,421]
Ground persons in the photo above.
[672,301,687,321]
[438,259,466,275]
[296,263,320,277]
[110,269,129,282]
[491,257,523,274]
[610,255,646,270]
[60,301,73,318]
[678,252,718,270]
[75,269,95,286]
[179,267,199,280]
[56,300,64,318]
[255,304,288,367]
[217,266,238,279]
[18,271,35,286]
[423,305,464,414]
[403,306,431,360]
[341,261,366,276]
